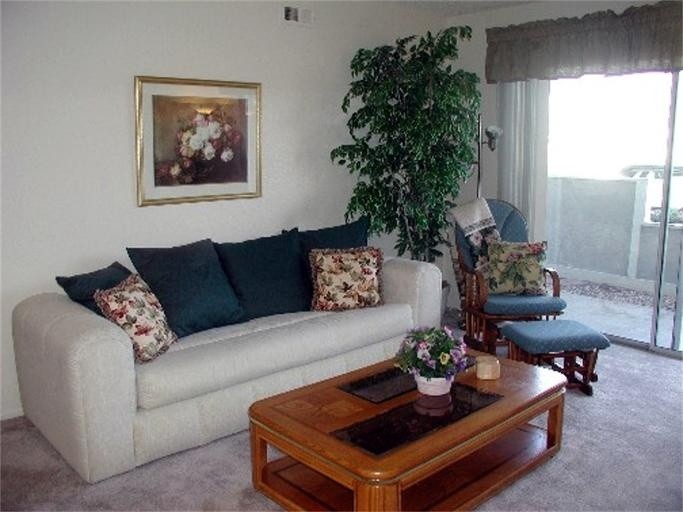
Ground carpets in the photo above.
[0,342,683,508]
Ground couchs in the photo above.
[13,257,442,484]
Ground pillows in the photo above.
[124,239,249,339]
[310,247,383,310]
[54,261,132,321]
[482,234,549,299]
[210,225,315,318]
[94,272,177,362]
[282,217,370,283]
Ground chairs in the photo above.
[453,197,565,355]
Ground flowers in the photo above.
[393,326,477,385]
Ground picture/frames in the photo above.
[136,77,262,206]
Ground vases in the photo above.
[414,374,453,396]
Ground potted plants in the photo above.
[331,27,482,317]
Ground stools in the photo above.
[503,319,610,397]
